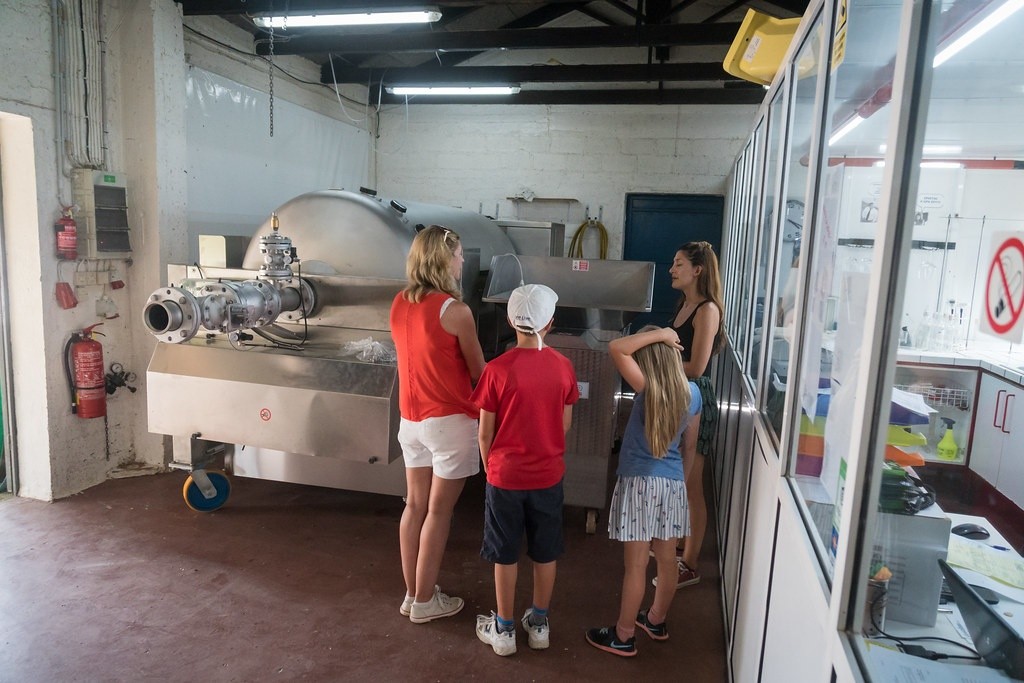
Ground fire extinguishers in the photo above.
[65,323,107,418]
[57,206,78,260]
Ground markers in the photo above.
[870,561,886,579]
[873,567,892,580]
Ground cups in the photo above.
[861,578,888,636]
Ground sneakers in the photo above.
[408,584,464,623]
[521,608,549,649]
[586,625,638,655]
[400,584,442,616]
[652,561,700,588]
[476,610,516,655]
[635,607,669,639]
[649,543,684,561]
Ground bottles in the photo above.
[901,325,909,348]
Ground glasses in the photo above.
[442,226,452,242]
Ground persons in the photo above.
[779,253,804,343]
[586,326,705,658]
[390,224,488,625]
[648,243,727,590]
[469,285,579,655]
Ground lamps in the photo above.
[251,3,445,29]
[383,80,526,99]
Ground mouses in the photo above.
[951,523,990,540]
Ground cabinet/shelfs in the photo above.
[794,361,1024,511]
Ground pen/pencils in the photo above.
[989,545,1010,550]
[937,608,953,613]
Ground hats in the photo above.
[507,283,559,351]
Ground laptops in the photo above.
[936,558,1024,679]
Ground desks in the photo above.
[864,512,1024,683]
